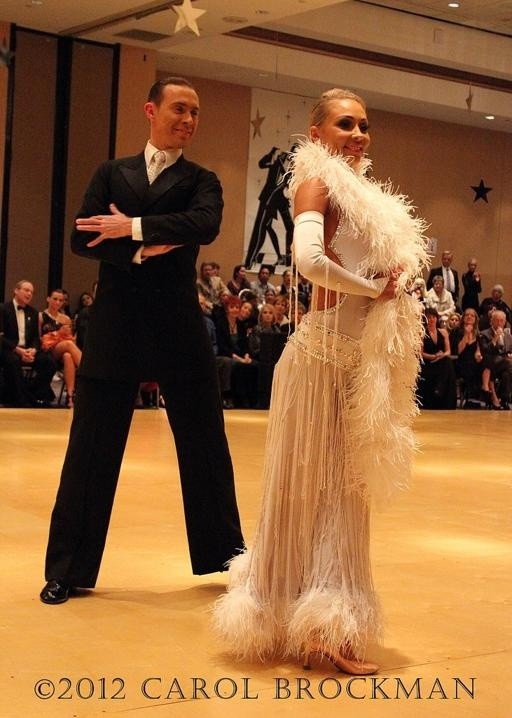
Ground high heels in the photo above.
[479,387,503,410]
[303,634,380,675]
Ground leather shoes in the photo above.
[40,576,74,604]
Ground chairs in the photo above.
[24,352,511,409]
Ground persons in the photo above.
[0,279,55,405]
[61,290,72,317]
[39,77,257,607]
[222,88,426,677]
[40,289,82,407]
[80,280,101,350]
[134,242,512,409]
[241,142,301,270]
[72,293,93,330]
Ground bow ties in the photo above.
[18,305,25,311]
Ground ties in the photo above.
[147,151,166,185]
[446,269,450,291]
